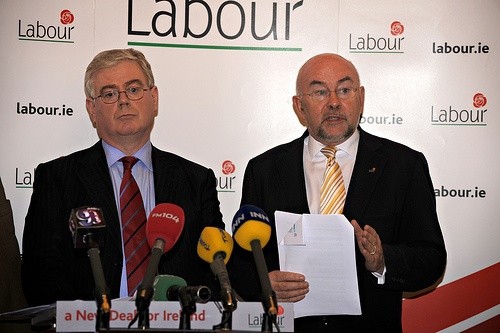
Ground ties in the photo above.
[119,156,154,296]
[318,146,346,214]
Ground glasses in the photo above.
[89,84,153,105]
[299,88,359,102]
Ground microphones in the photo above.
[197,227,235,308]
[68,206,113,314]
[232,204,278,318]
[137,203,185,308]
[153,275,211,303]
